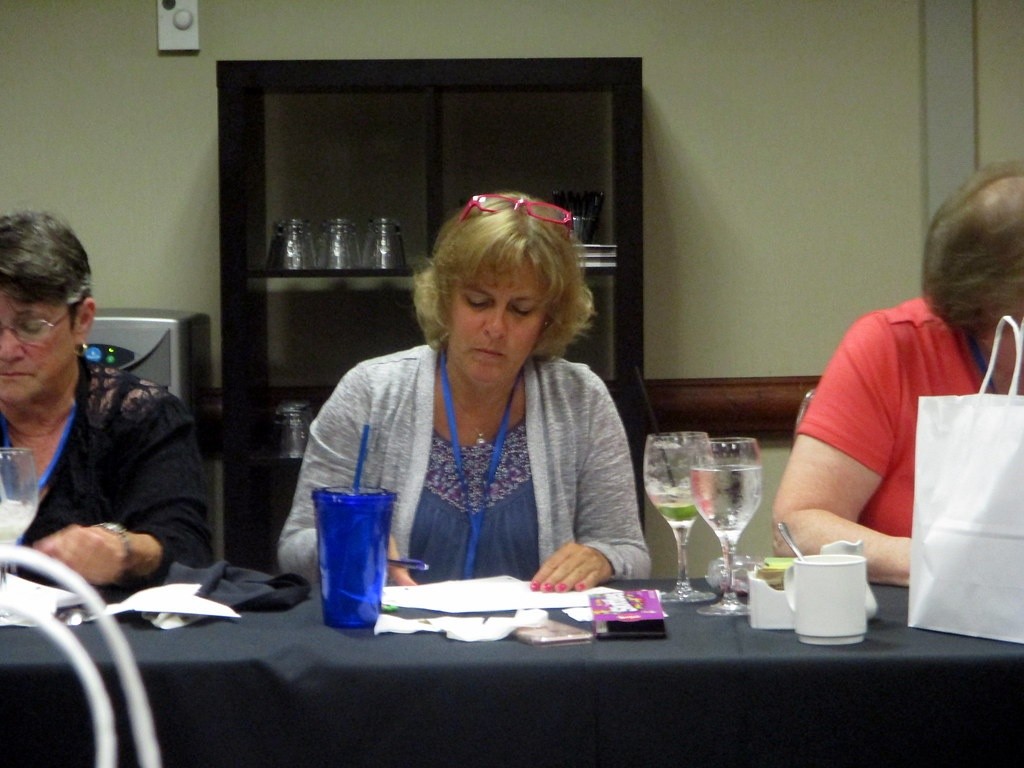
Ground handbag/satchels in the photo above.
[908,315,1023,645]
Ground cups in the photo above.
[265,217,314,270]
[362,217,406,269]
[314,219,362,272]
[311,486,396,628]
[784,555,867,645]
[275,400,312,458]
[574,216,597,244]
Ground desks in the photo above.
[0,577,1024,768]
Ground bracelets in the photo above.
[91,522,131,552]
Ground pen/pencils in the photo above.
[387,557,430,570]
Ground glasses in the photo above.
[459,194,574,240]
[0,309,73,347]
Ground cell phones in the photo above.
[511,620,594,647]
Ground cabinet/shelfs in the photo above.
[214,59,642,580]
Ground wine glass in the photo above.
[643,431,716,602]
[0,448,38,618]
[690,437,763,617]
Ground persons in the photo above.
[770,160,1024,588]
[0,210,208,606]
[277,191,652,594]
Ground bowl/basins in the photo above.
[706,554,763,592]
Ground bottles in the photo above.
[820,540,877,620]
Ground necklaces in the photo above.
[453,400,503,445]
